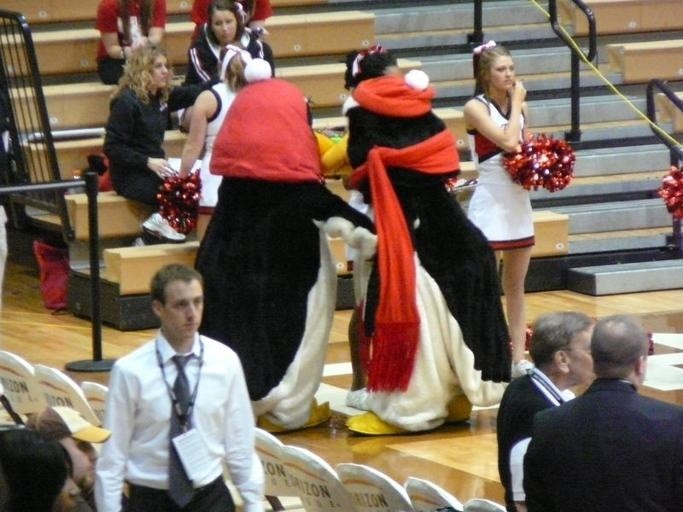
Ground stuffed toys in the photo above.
[320,69,512,437]
[194,78,379,434]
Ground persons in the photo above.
[27,406,112,512]
[103,43,220,246]
[0,428,82,511]
[95,0,167,84]
[463,40,536,380]
[94,263,269,512]
[177,1,277,129]
[180,44,253,245]
[190,0,273,41]
[495,311,594,509]
[343,45,402,409]
[521,313,683,509]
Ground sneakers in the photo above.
[510,357,535,380]
[140,213,185,243]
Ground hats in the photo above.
[26,405,111,443]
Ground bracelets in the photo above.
[118,44,127,61]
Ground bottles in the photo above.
[67,169,86,194]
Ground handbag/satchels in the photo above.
[32,237,68,310]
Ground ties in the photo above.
[168,354,195,508]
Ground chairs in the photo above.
[253,427,298,496]
[0,351,39,415]
[280,446,351,512]
[335,463,415,512]
[404,477,463,512]
[81,382,112,427]
[464,498,504,512]
[33,363,99,427]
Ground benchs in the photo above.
[654,93,683,134]
[64,163,477,246]
[553,2,683,38]
[106,210,567,297]
[24,112,463,182]
[7,55,419,132]
[1,12,375,81]
[0,0,326,24]
[608,39,683,84]
